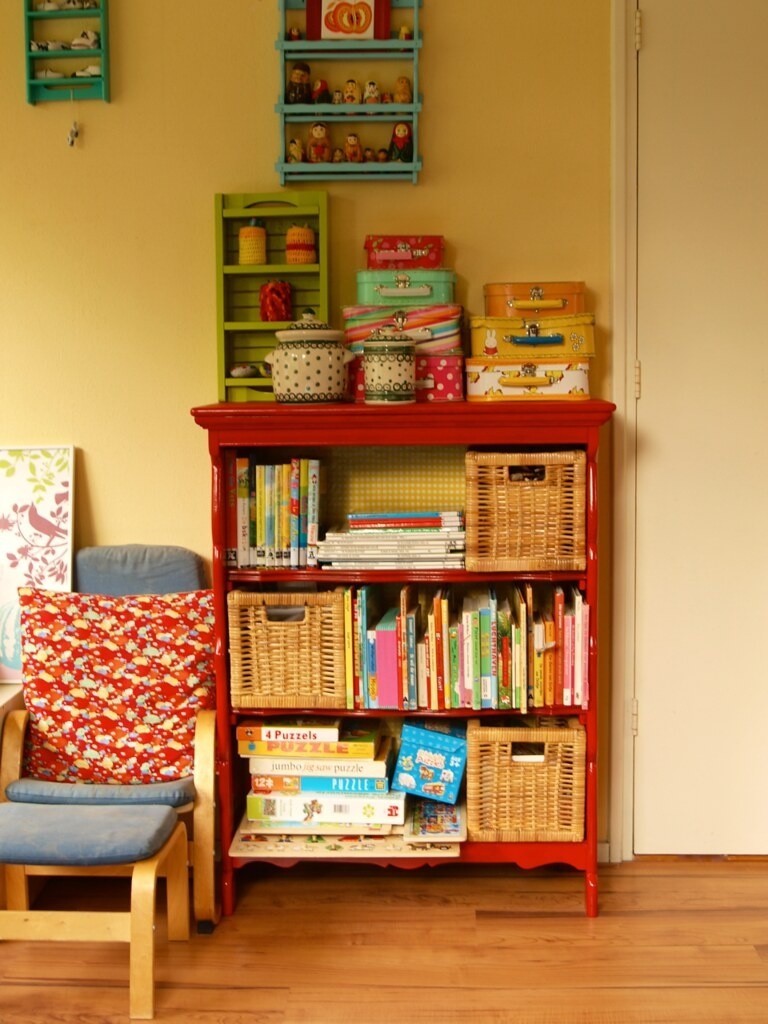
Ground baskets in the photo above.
[463,449,587,573]
[226,584,347,712]
[465,718,587,844]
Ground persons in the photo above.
[295,309,323,324]
[284,24,414,163]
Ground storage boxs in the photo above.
[341,234,463,402]
[391,717,468,805]
[464,282,596,401]
[236,717,407,825]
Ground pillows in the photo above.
[15,586,215,786]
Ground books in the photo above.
[235,457,590,825]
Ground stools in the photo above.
[0,800,190,1019]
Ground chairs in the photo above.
[0,543,224,935]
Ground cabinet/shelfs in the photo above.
[24,0,111,106]
[213,191,328,403]
[273,0,424,186]
[191,397,617,919]
